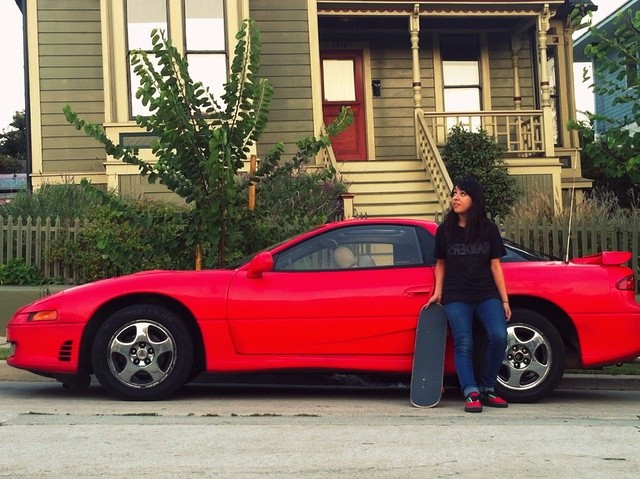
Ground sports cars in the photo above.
[6,218,640,401]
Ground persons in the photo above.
[422,179,512,413]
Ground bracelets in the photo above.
[503,301,508,303]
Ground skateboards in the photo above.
[409,299,448,409]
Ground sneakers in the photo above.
[465,392,481,412]
[481,391,508,407]
